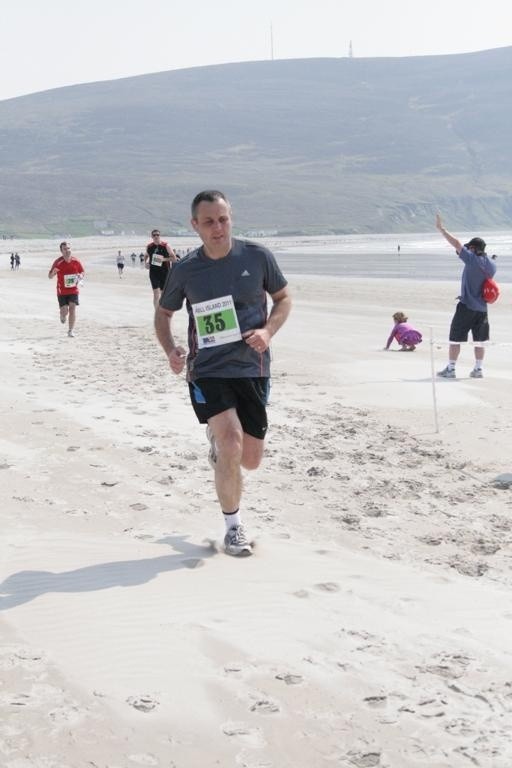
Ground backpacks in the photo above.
[482,272,500,304]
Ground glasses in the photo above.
[153,234,159,236]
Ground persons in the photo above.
[144,229,178,311]
[130,247,197,263]
[45,240,84,337]
[435,214,497,382]
[382,311,423,352]
[116,249,126,279]
[154,188,291,562]
[15,253,22,269]
[10,253,16,269]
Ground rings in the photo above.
[256,343,263,353]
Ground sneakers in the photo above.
[470,368,482,377]
[206,425,215,468]
[437,367,456,378]
[224,525,252,557]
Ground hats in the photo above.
[464,237,485,249]
